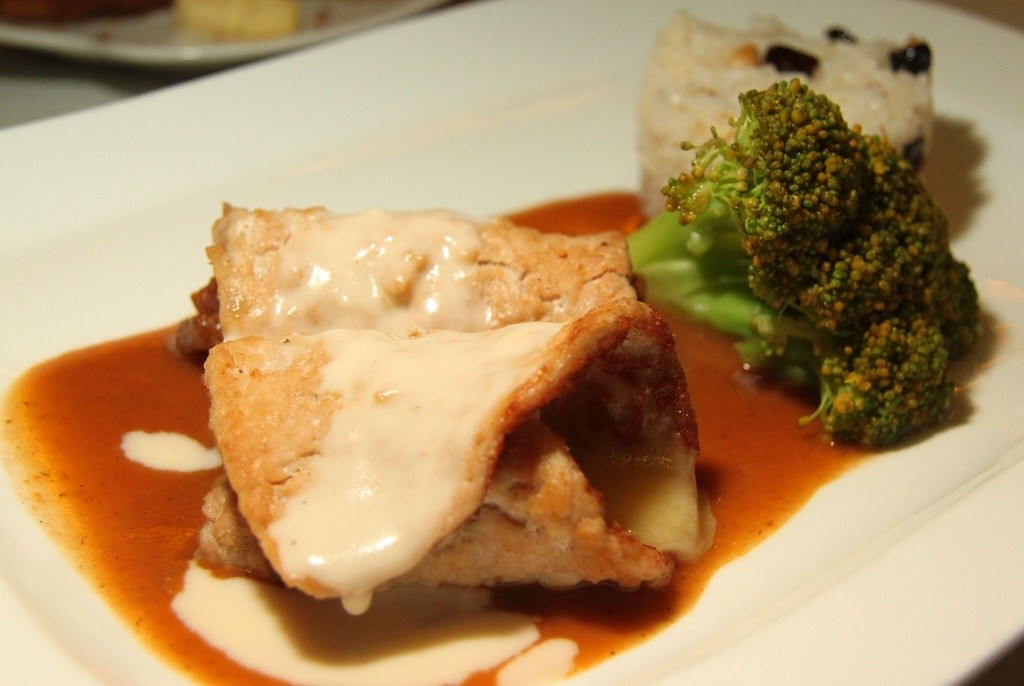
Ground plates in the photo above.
[0,0,453,73]
[0,0,1024,686]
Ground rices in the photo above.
[635,12,934,215]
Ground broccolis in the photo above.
[624,78,982,448]
